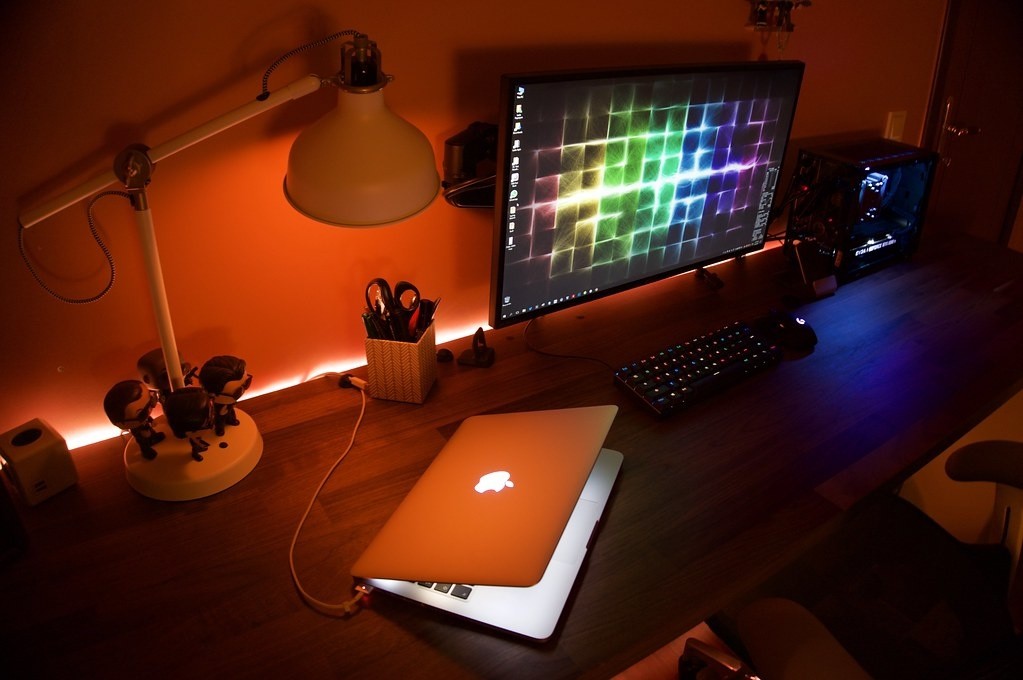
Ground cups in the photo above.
[366,320,436,405]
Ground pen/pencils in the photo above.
[362,278,441,343]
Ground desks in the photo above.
[0,218,1023,679]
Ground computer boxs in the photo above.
[784,134,939,276]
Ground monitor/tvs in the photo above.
[488,59,806,329]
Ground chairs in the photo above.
[678,439,1023,678]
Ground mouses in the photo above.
[762,312,818,349]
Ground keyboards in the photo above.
[612,319,778,416]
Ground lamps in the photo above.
[18,30,441,502]
